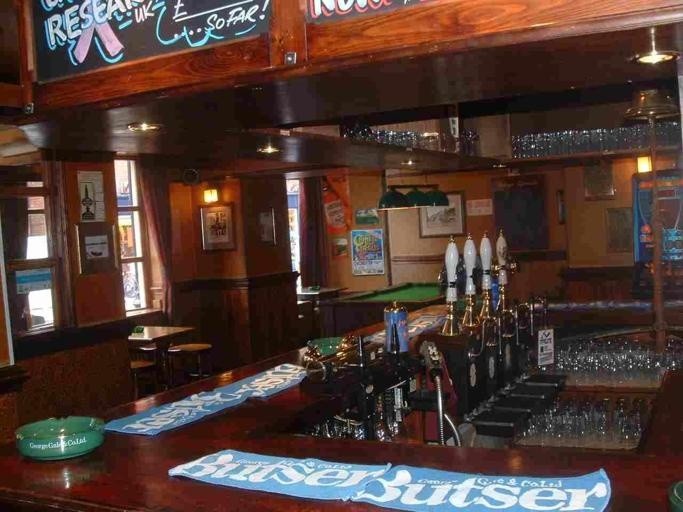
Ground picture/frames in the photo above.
[417,189,468,239]
[257,205,276,245]
[200,202,237,252]
[581,158,615,203]
[74,220,118,274]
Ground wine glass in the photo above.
[554,336,682,384]
[524,392,643,448]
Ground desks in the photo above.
[315,282,446,337]
[130,324,195,390]
[297,286,347,318]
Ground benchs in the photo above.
[167,342,213,381]
[131,360,159,400]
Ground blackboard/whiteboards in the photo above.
[490,174,548,249]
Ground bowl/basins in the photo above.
[14,416,105,461]
[308,335,342,354]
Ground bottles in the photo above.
[346,125,441,152]
[467,130,478,157]
[512,123,678,162]
[315,390,416,443]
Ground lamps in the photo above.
[400,147,418,166]
[127,120,161,134]
[376,185,450,212]
[201,179,220,205]
[625,25,682,70]
[636,156,653,174]
[256,135,281,156]
